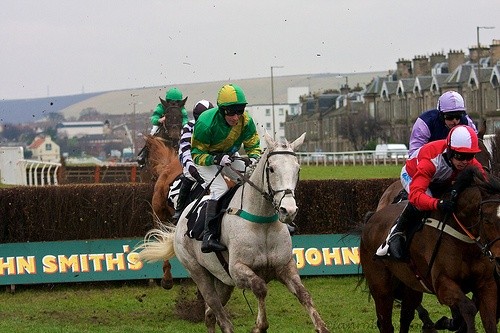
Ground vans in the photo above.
[376,143,409,158]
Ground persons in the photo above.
[389,91,489,262]
[139,83,264,254]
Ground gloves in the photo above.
[437,200,456,212]
[219,155,257,168]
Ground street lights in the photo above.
[335,75,353,153]
[476,25,495,115]
[270,66,285,147]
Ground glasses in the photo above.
[222,107,245,115]
[443,112,462,121]
[455,152,475,161]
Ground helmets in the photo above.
[193,100,214,115]
[437,91,465,113]
[217,84,248,107]
[447,125,481,153]
[166,87,182,100]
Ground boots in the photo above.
[139,143,152,165]
[173,182,191,220]
[201,199,227,254]
[387,201,426,261]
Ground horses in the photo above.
[137,96,188,164]
[376,127,500,214]
[144,135,236,314]
[354,174,500,333]
[132,129,329,333]
